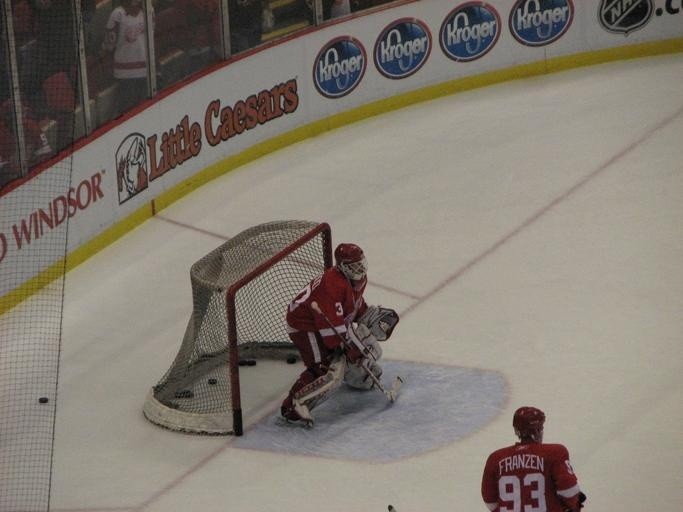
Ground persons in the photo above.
[229,1,353,54]
[2,1,156,183]
[277,243,398,427]
[479,407,584,511]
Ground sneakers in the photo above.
[280,401,314,423]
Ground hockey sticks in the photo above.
[312,302,403,403]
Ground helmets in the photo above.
[335,243,368,280]
[513,407,545,437]
